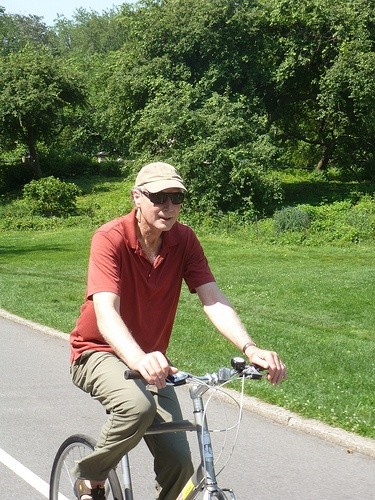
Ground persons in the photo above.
[66,161,285,500]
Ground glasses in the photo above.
[141,191,184,204]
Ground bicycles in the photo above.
[49,356,270,499]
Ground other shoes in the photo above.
[73,478,106,500]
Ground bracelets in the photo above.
[242,342,256,357]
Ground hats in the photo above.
[135,162,187,194]
